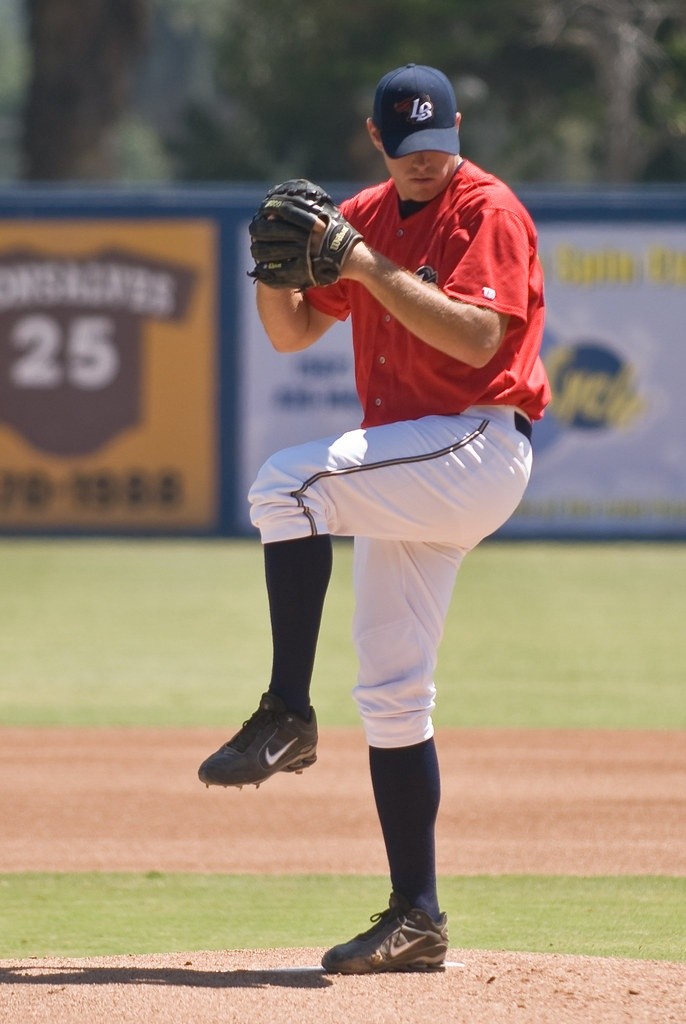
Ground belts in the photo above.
[514,411,533,441]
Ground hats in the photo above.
[372,63,460,159]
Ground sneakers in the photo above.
[198,692,318,790]
[321,892,449,974]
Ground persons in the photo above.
[197,65,549,970]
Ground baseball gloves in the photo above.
[242,174,367,295]
